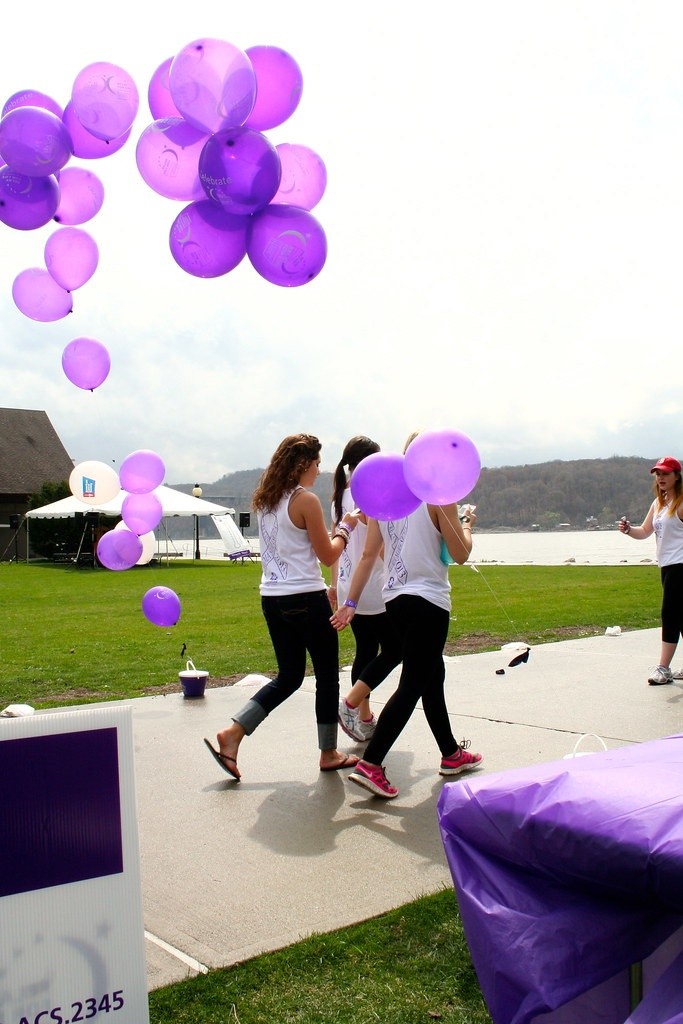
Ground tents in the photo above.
[25,474,236,564]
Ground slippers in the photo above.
[320,753,359,772]
[204,738,241,782]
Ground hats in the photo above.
[650,457,681,473]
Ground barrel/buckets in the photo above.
[501,641,530,667]
[178,660,209,697]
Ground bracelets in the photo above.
[332,522,351,549]
[329,585,336,590]
[463,527,472,530]
[344,600,357,609]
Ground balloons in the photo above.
[97,530,143,571]
[122,488,163,535]
[12,227,98,322]
[403,428,482,505]
[349,449,421,522]
[69,462,121,505]
[136,37,329,286]
[113,518,154,565]
[142,587,181,626]
[62,337,110,390]
[0,62,139,230]
[119,449,165,494]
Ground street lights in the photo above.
[191,484,203,559]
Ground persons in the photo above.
[618,457,683,684]
[327,437,384,740]
[328,429,484,798]
[204,433,360,781]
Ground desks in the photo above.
[436,735,683,1024]
[54,552,93,566]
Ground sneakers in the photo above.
[348,760,399,798]
[438,740,484,775]
[357,712,379,739]
[648,666,673,684]
[672,668,683,678]
[338,698,366,742]
